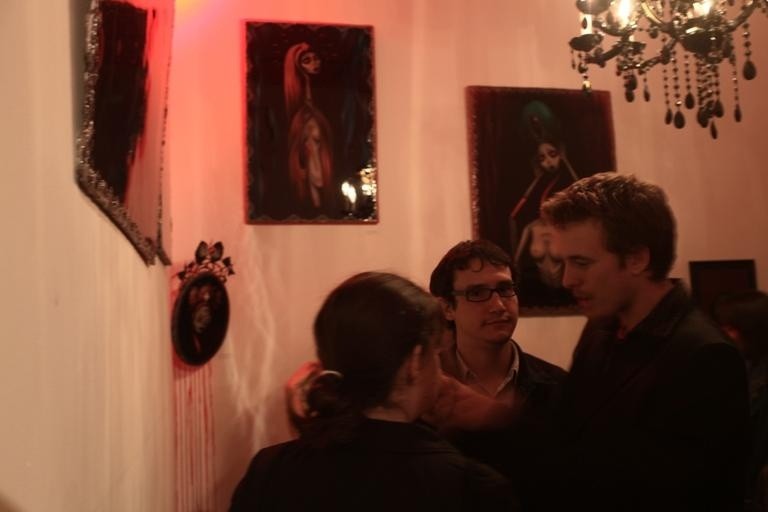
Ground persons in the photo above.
[176,284,225,363]
[284,41,334,219]
[229,270,541,511]
[713,288,767,431]
[509,100,589,291]
[429,240,564,471]
[426,170,768,512]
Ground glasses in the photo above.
[450,284,518,302]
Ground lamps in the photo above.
[570,1,768,139]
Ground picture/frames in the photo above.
[241,19,380,225]
[76,1,175,267]
[466,86,617,316]
[689,260,756,304]
[171,242,235,365]
